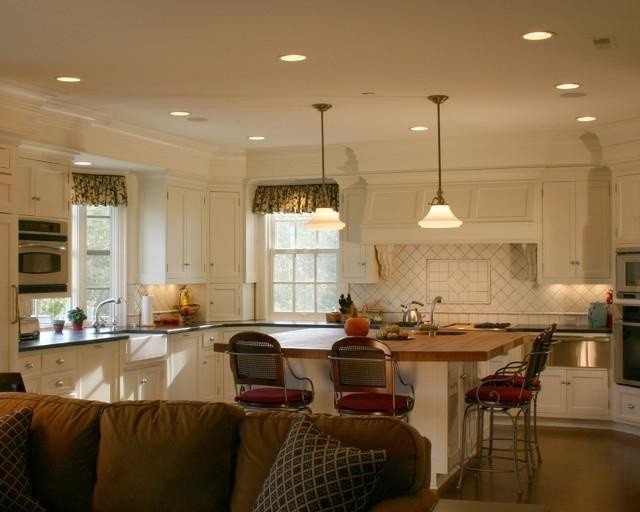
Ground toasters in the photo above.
[19,317,40,340]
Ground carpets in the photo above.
[433,498,545,512]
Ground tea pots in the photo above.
[400,301,424,323]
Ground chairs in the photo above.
[231,331,414,422]
[457,323,556,493]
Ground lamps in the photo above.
[418,94,461,227]
[305,102,345,232]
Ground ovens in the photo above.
[613,300,640,389]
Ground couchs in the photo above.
[0,390,439,512]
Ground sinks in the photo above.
[102,329,165,337]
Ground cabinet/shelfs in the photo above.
[0,143,20,372]
[611,156,640,254]
[204,283,256,327]
[139,170,207,284]
[207,184,256,280]
[119,360,167,401]
[341,186,376,284]
[527,367,611,418]
[19,152,69,219]
[20,342,118,404]
[167,322,258,403]
[536,168,611,285]
[607,384,639,427]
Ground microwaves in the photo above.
[615,247,640,299]
[19,230,69,297]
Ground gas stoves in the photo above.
[474,322,510,329]
[389,320,429,328]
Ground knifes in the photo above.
[338,293,352,314]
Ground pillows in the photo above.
[0,407,46,512]
[250,414,385,512]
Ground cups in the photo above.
[52,319,65,333]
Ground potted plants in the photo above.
[69,308,86,329]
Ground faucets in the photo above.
[431,296,444,322]
[92,296,122,332]
